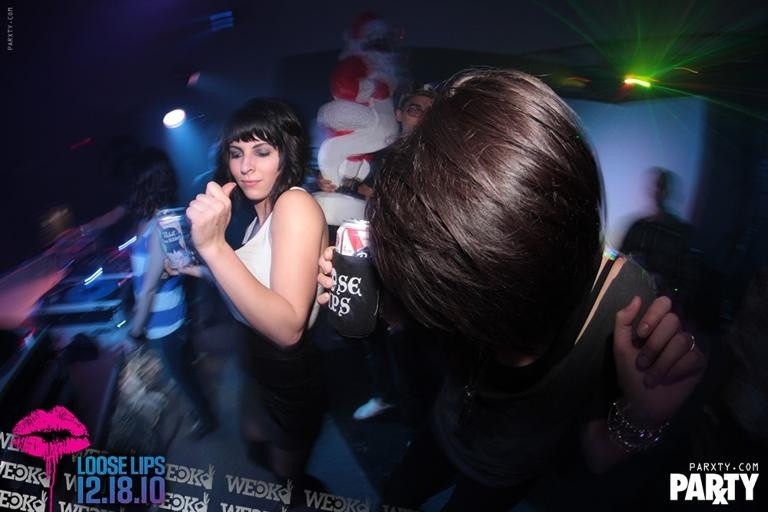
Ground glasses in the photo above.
[399,105,424,118]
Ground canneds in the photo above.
[155,215,190,271]
[335,216,381,339]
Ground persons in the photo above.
[0,73,766,509]
